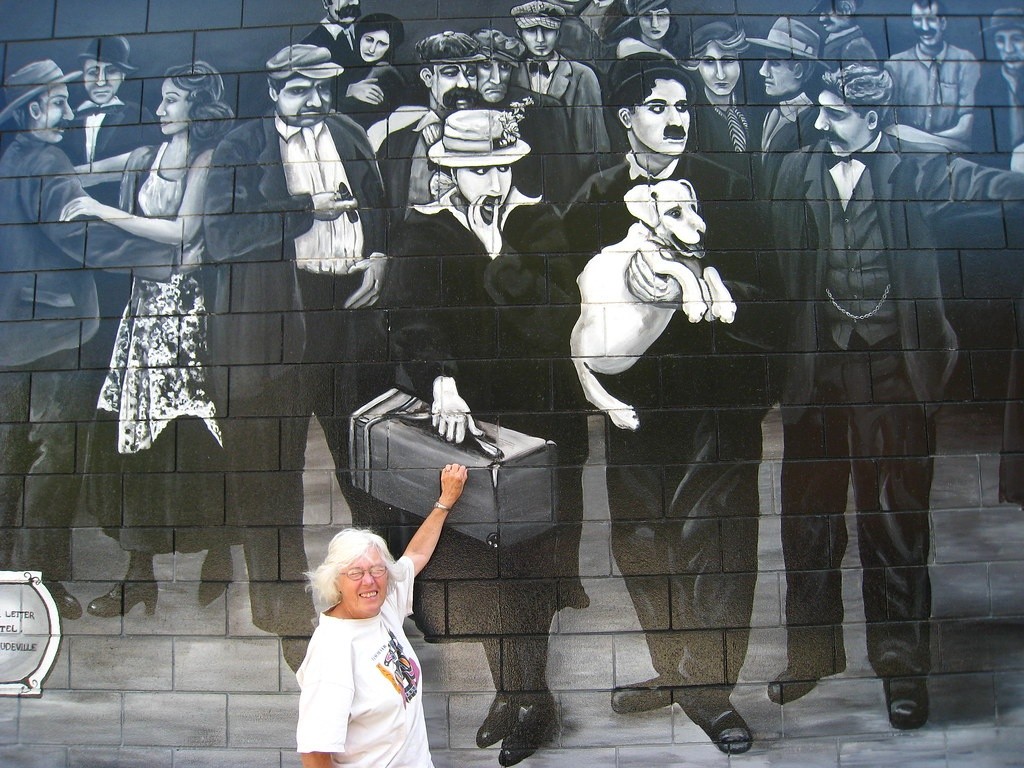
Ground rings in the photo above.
[445,469,451,472]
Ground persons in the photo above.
[296,464,468,768]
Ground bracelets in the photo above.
[434,502,451,511]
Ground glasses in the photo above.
[338,565,389,581]
